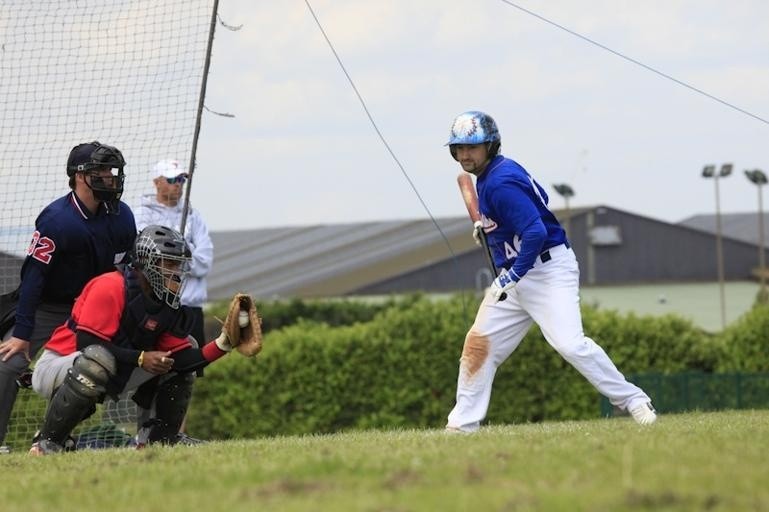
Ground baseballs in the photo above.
[238,311,249,327]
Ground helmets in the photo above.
[443,111,501,146]
[67,141,126,172]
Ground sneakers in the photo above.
[170,433,202,445]
[632,401,657,427]
[30,431,63,454]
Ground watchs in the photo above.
[137,350,145,370]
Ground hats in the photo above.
[134,225,192,260]
[151,158,190,178]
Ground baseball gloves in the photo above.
[213,294,262,357]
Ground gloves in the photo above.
[490,267,520,305]
[473,221,483,247]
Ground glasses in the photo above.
[168,178,185,184]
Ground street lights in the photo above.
[701,164,733,329]
[552,183,576,240]
[744,169,768,268]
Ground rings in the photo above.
[161,356,166,363]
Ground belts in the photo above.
[541,242,570,262]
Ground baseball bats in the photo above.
[457,174,508,302]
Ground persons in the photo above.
[26,227,261,455]
[1,142,137,456]
[445,113,658,432]
[134,158,213,447]
